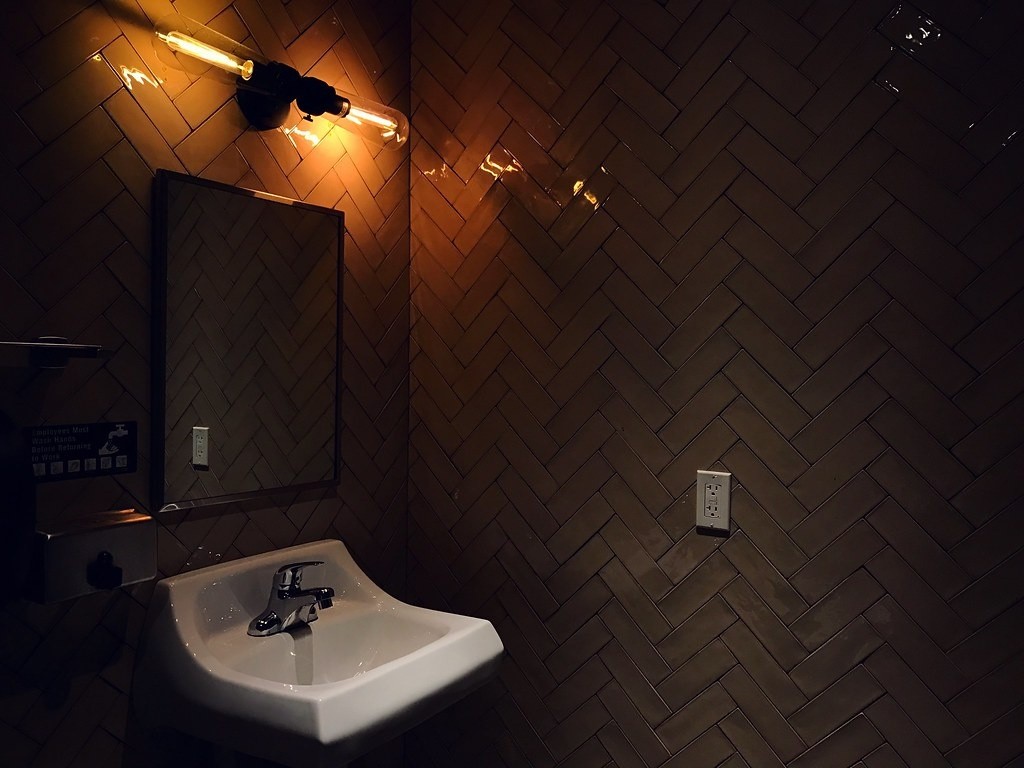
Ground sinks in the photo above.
[205,597,507,745]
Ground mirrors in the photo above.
[148,165,346,518]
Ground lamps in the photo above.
[150,11,411,154]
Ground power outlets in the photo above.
[696,470,732,531]
[193,427,210,466]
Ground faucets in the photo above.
[246,560,336,637]
